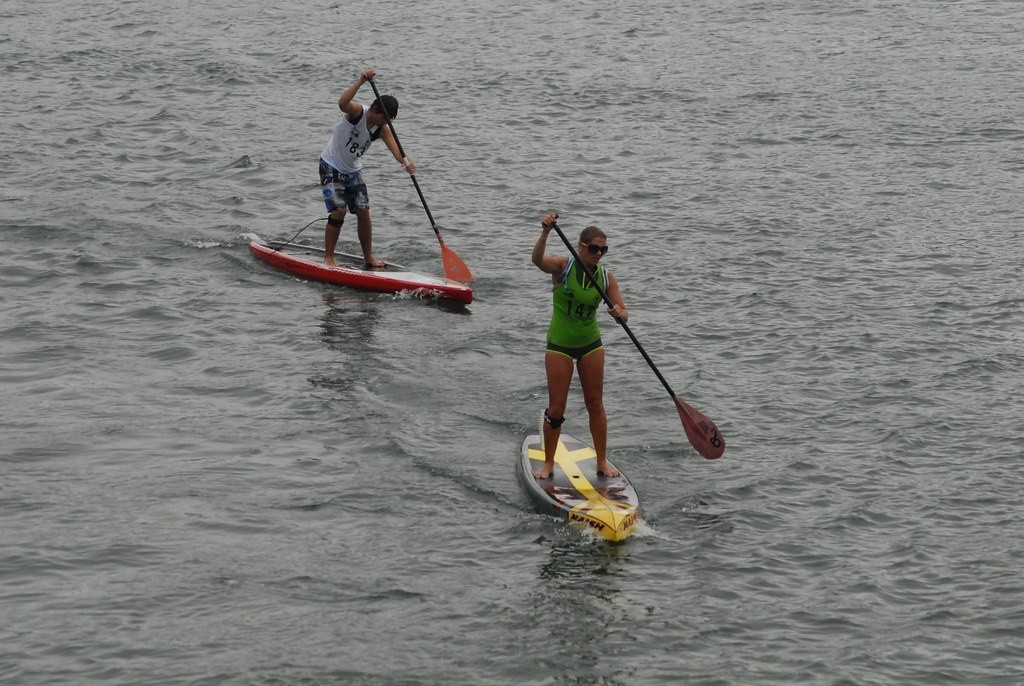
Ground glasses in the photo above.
[581,242,609,254]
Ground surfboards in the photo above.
[516,432,642,542]
[246,237,474,307]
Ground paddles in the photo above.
[540,213,728,462]
[362,73,473,285]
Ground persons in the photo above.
[531,213,628,478]
[318,67,415,269]
